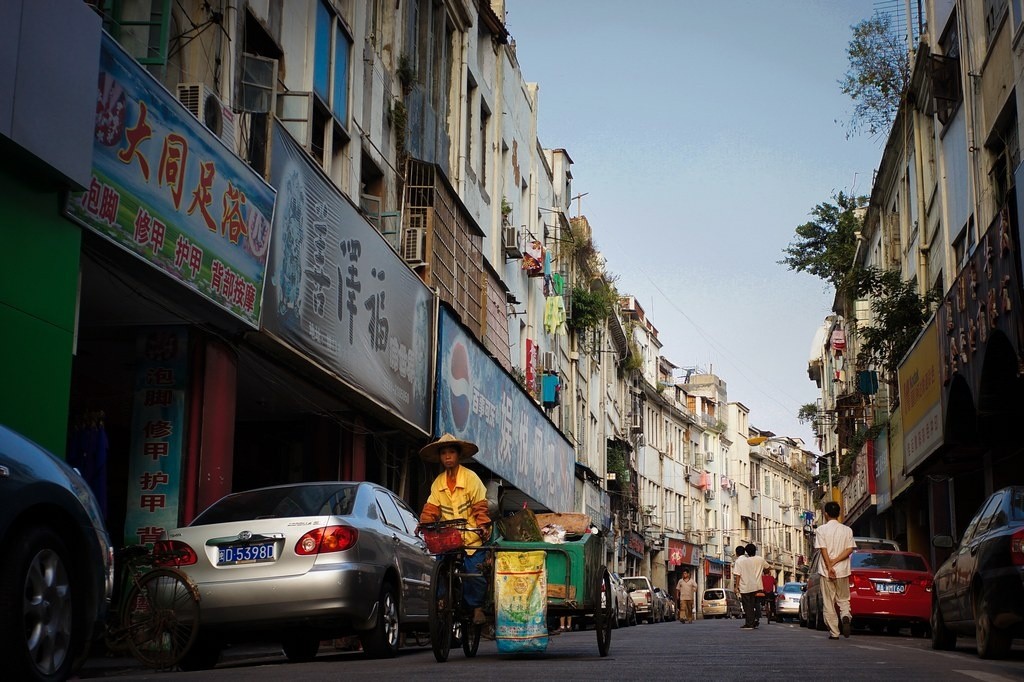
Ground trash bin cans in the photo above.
[494,511,600,611]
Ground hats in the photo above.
[420,433,479,460]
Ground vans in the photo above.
[702,587,743,619]
[797,536,906,628]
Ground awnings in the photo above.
[705,555,732,565]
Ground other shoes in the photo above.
[740,624,754,630]
[473,612,486,625]
[679,618,685,624]
[842,616,851,638]
[829,636,839,640]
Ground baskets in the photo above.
[417,518,468,556]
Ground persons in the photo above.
[761,569,778,618]
[735,546,748,574]
[736,543,772,629]
[676,569,698,624]
[413,431,493,625]
[815,502,858,641]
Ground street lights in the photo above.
[746,436,832,503]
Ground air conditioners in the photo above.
[698,535,706,546]
[723,536,731,546]
[751,489,758,497]
[543,351,557,375]
[706,489,714,500]
[707,527,716,537]
[706,451,714,462]
[640,436,646,447]
[777,548,782,555]
[176,82,235,152]
[503,225,524,259]
[404,227,426,264]
[644,516,653,527]
[766,544,773,554]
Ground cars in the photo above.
[0,423,114,682]
[570,570,676,631]
[147,480,465,672]
[932,486,1024,659]
[799,550,935,639]
[774,582,808,623]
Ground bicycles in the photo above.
[114,545,203,675]
[764,597,772,625]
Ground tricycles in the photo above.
[413,512,615,664]
